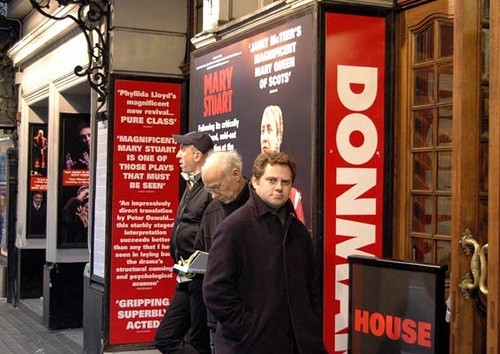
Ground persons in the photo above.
[28,127,91,242]
[261,105,305,226]
[191,151,252,354]
[203,153,324,354]
[154,131,214,354]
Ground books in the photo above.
[173,250,209,274]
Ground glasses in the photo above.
[204,176,229,193]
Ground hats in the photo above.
[170,132,214,151]
